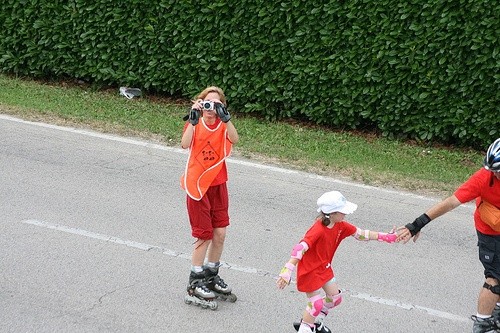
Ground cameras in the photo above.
[199,100,214,110]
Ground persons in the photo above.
[397,138,500,333]
[276,190,405,333]
[180,87,238,310]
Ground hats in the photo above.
[317,191,358,214]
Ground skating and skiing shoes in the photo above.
[184,270,218,311]
[201,262,237,303]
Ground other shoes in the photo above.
[297,323,316,333]
[471,315,500,333]
[491,303,500,322]
[293,321,331,333]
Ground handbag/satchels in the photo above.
[479,202,500,232]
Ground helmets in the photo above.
[483,138,500,171]
